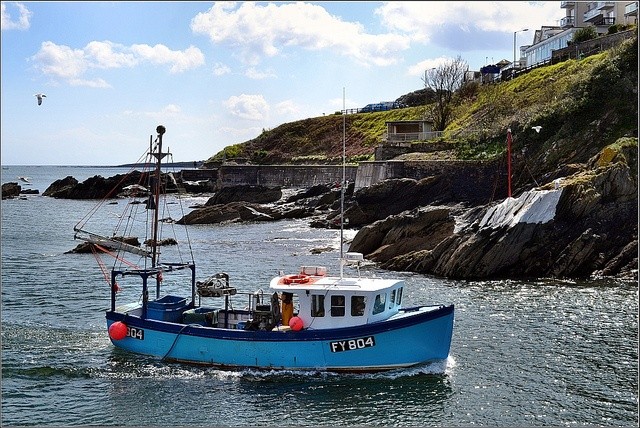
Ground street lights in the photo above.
[424,67,436,89]
[513,28,529,68]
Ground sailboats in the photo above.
[66,126,455,372]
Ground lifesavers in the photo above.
[283,275,311,284]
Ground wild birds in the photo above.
[243,204,274,219]
[182,179,210,186]
[531,125,544,133]
[16,175,32,183]
[34,93,47,105]
